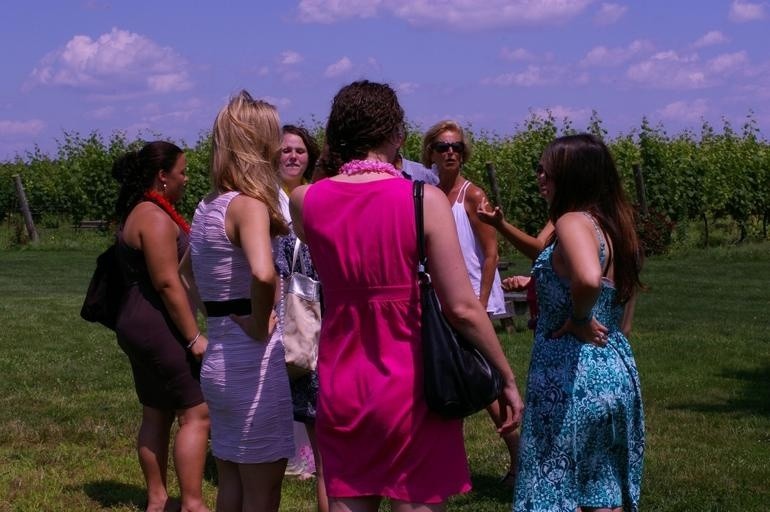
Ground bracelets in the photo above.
[186,331,201,349]
[315,157,338,174]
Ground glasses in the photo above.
[431,141,465,153]
[537,164,543,175]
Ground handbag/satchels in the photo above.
[81,240,131,332]
[283,272,322,371]
[419,282,503,420]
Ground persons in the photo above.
[476,132,644,512]
[421,120,524,492]
[287,78,525,512]
[268,125,331,512]
[188,89,297,512]
[394,123,442,190]
[110,140,213,512]
